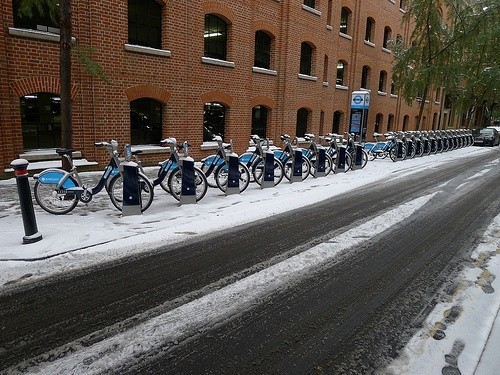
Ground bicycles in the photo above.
[365,128,474,162]
[158,134,251,194]
[33,140,154,215]
[201,133,368,188]
[106,137,207,202]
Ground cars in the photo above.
[472,126,500,147]
